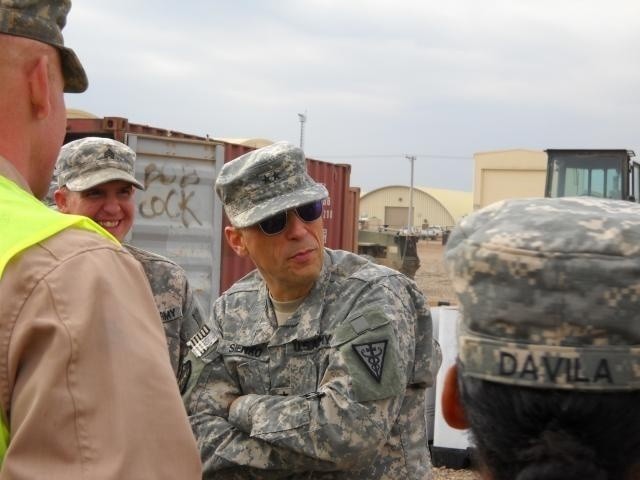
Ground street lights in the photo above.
[297,111,307,149]
[405,155,417,229]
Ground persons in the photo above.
[441,198,640,480]
[53,137,205,447]
[188,142,440,480]
[0,0,204,480]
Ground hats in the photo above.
[55,136,144,193]
[215,142,328,229]
[442,197,639,393]
[1,0,88,93]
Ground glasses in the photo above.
[257,200,324,236]
